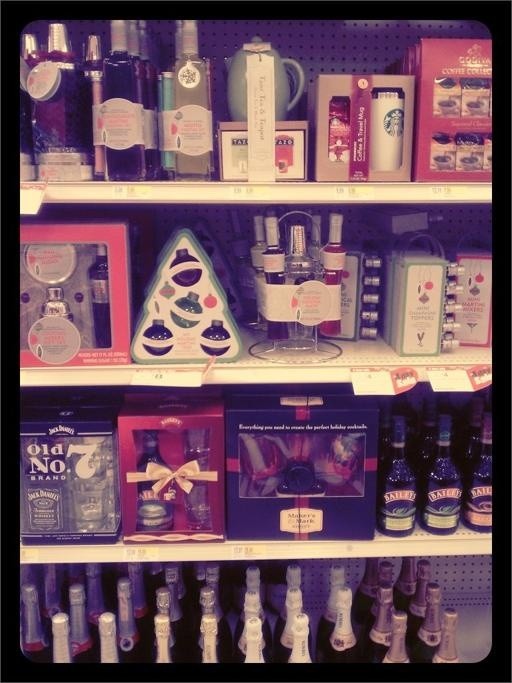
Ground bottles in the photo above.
[26,388,493,535]
[18,558,459,663]
[20,20,212,181]
[21,209,463,357]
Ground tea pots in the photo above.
[224,37,306,120]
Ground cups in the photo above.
[434,97,485,172]
[368,96,404,173]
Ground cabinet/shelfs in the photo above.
[20,18,493,665]
